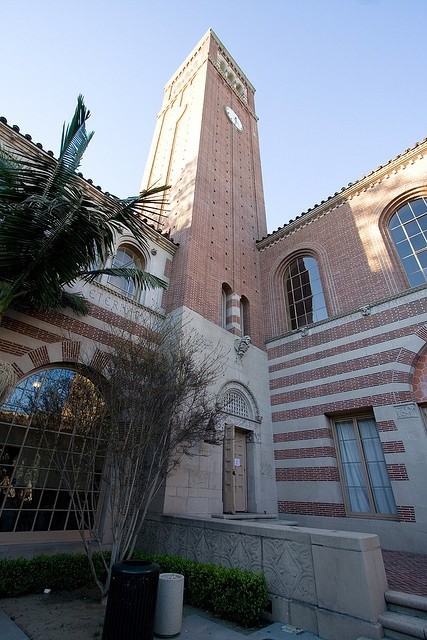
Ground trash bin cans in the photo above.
[102,559,160,640]
